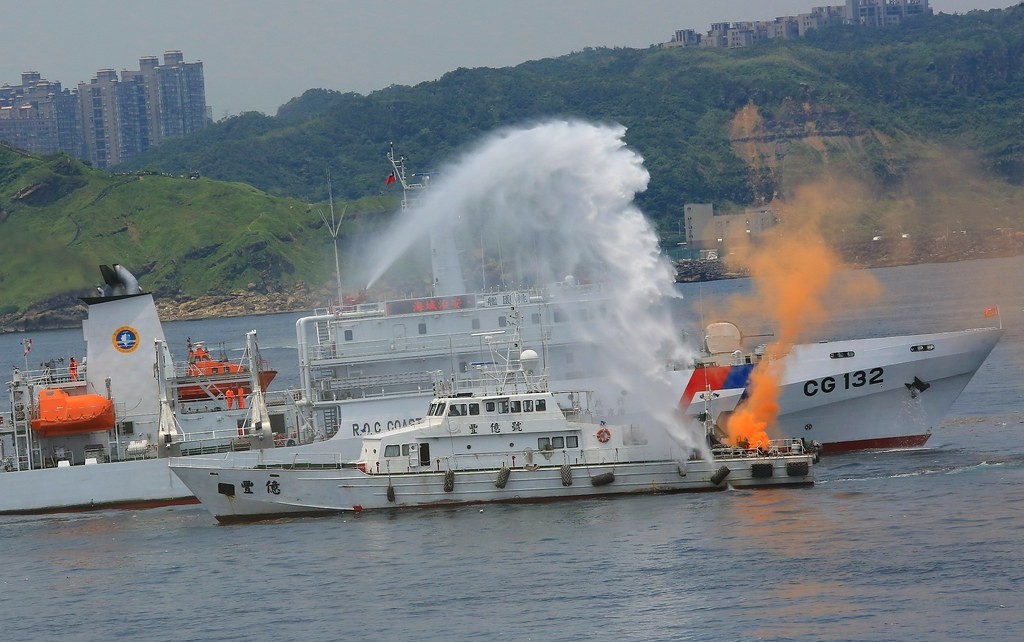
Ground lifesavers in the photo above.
[596,427,611,443]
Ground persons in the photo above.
[238,385,245,409]
[69,356,78,381]
[226,387,235,409]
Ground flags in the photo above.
[983,304,999,317]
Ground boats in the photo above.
[0,141,1007,526]
